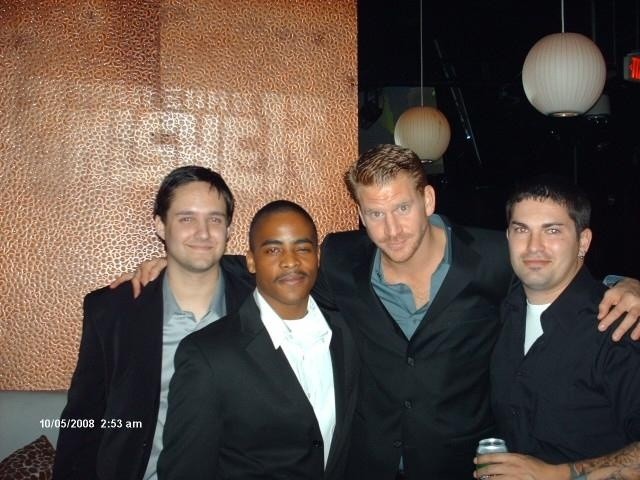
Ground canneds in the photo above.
[476,438,509,480]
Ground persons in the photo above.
[51,162,256,480]
[109,140,640,480]
[153,197,359,479]
[471,170,640,479]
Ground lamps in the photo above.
[394,1,451,163]
[521,1,607,117]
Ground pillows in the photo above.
[1,436,56,478]
